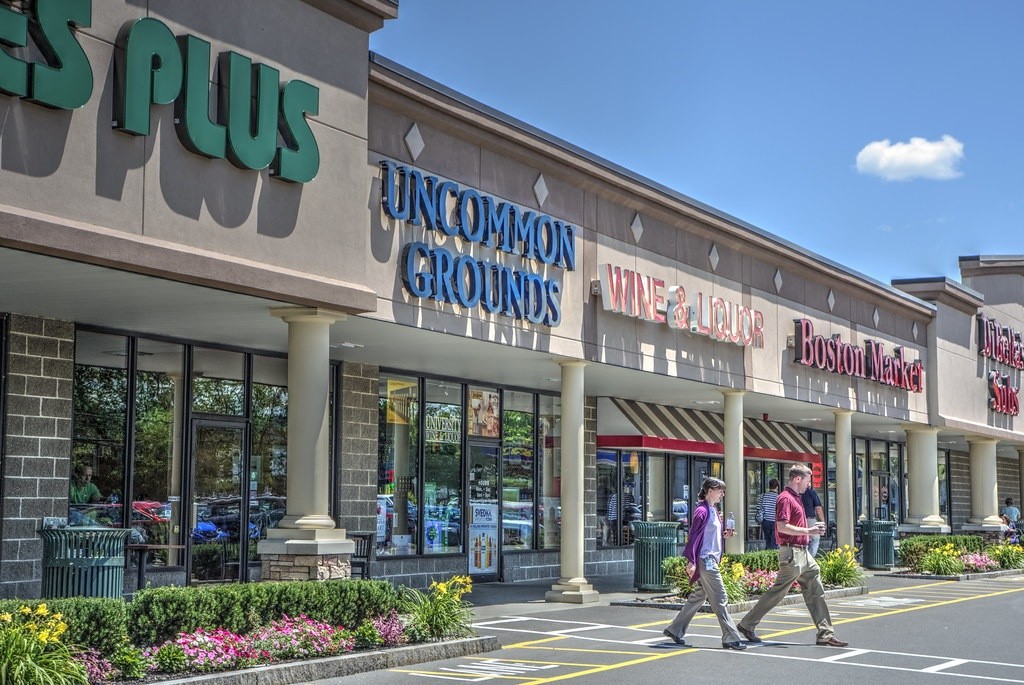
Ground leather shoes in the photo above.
[737,624,761,642]
[816,637,849,646]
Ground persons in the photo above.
[68,462,121,505]
[799,482,827,558]
[663,477,747,650]
[999,497,1021,537]
[758,479,780,550]
[736,463,848,647]
[597,477,633,546]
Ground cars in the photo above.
[377,494,461,547]
[503,501,543,548]
[67,494,287,548]
[674,499,687,520]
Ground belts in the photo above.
[780,543,807,550]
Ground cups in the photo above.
[815,522,826,535]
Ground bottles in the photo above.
[727,512,735,536]
[487,394,494,434]
[471,533,497,570]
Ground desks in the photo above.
[128,544,185,592]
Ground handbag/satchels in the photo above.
[755,493,765,524]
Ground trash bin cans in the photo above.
[35,526,133,601]
[629,521,681,590]
[859,520,896,571]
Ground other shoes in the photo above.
[663,629,686,645]
[723,639,746,649]
